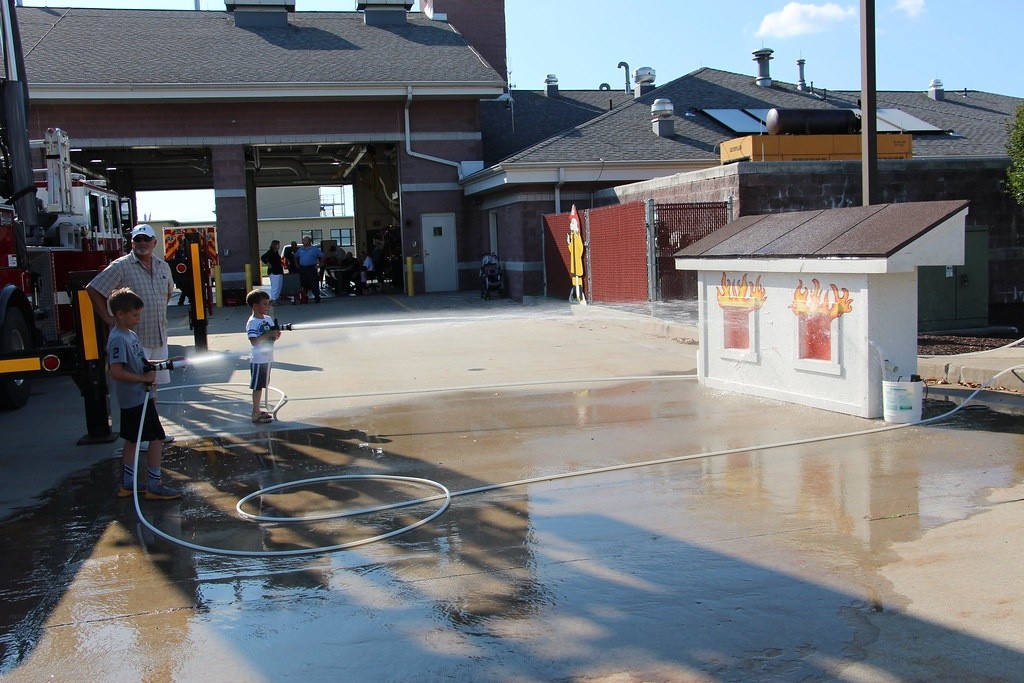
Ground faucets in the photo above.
[270,318,292,331]
[142,358,174,393]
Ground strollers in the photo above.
[480,252,508,300]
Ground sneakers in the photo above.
[138,439,149,451]
[116,477,147,497]
[144,480,184,500]
[162,433,174,443]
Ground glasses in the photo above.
[132,235,153,242]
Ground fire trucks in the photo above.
[0,0,138,410]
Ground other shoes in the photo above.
[251,411,273,424]
[314,295,321,303]
[271,301,281,306]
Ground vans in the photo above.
[281,244,320,274]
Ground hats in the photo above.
[129,223,155,240]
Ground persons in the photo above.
[246,289,281,424]
[85,224,175,451]
[261,240,283,300]
[324,246,375,290]
[286,241,300,274]
[294,237,324,304]
[106,288,183,499]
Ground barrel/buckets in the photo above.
[881,376,923,423]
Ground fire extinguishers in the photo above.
[297,288,307,303]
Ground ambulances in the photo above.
[162,226,218,287]
